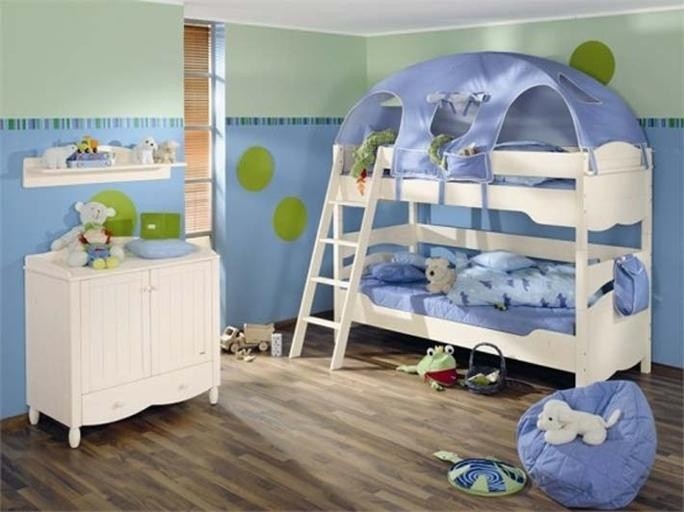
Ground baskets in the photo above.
[465,343,506,395]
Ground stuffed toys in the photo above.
[66,134,116,170]
[395,345,467,392]
[349,131,452,180]
[536,399,621,446]
[50,202,125,270]
[129,139,177,165]
[424,258,457,294]
[40,144,77,170]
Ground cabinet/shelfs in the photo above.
[24,235,221,450]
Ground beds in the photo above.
[331,142,653,388]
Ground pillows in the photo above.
[372,251,536,283]
